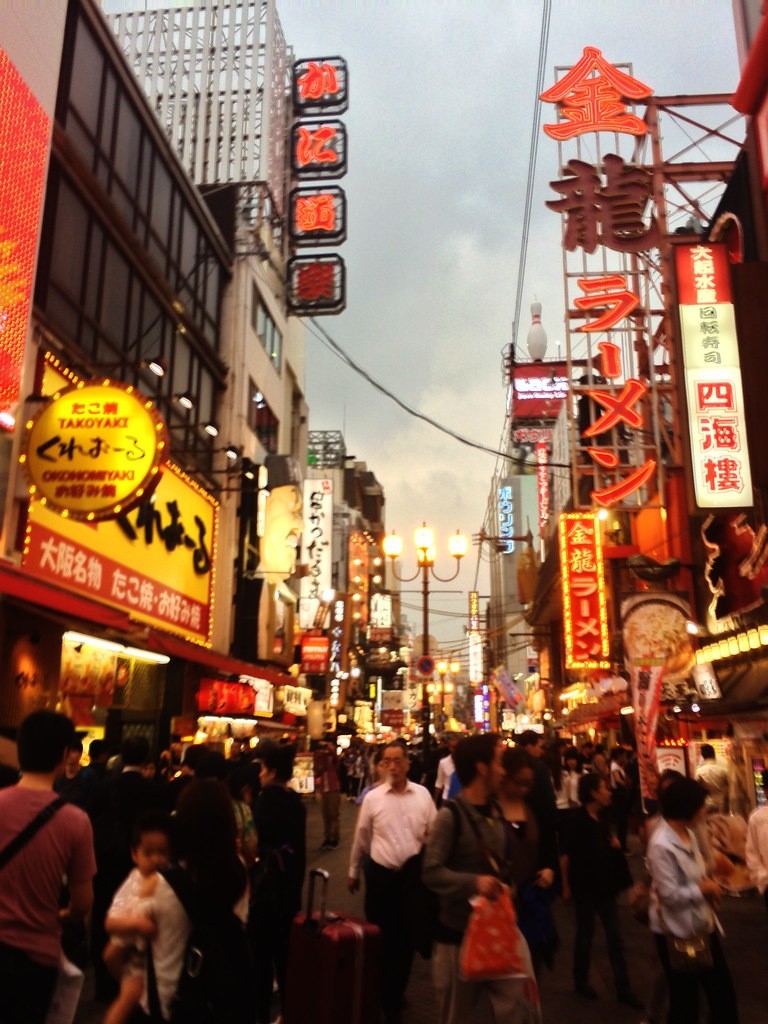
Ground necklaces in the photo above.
[506,803,523,828]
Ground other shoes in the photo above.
[327,840,340,850]
[576,983,596,1000]
[319,840,329,849]
[621,850,633,858]
[620,993,645,1009]
[346,796,355,802]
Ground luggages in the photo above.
[281,868,379,1024]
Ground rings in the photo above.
[543,885,546,888]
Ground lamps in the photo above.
[224,445,239,460]
[201,420,219,437]
[176,390,195,410]
[145,358,168,377]
[242,456,256,480]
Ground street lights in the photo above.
[384,520,470,792]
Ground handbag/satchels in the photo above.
[627,882,652,923]
[459,882,529,984]
[668,936,710,977]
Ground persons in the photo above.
[421,730,646,1024]
[54,726,308,1024]
[348,739,441,1024]
[639,743,768,1024]
[0,710,98,1024]
[314,732,476,852]
